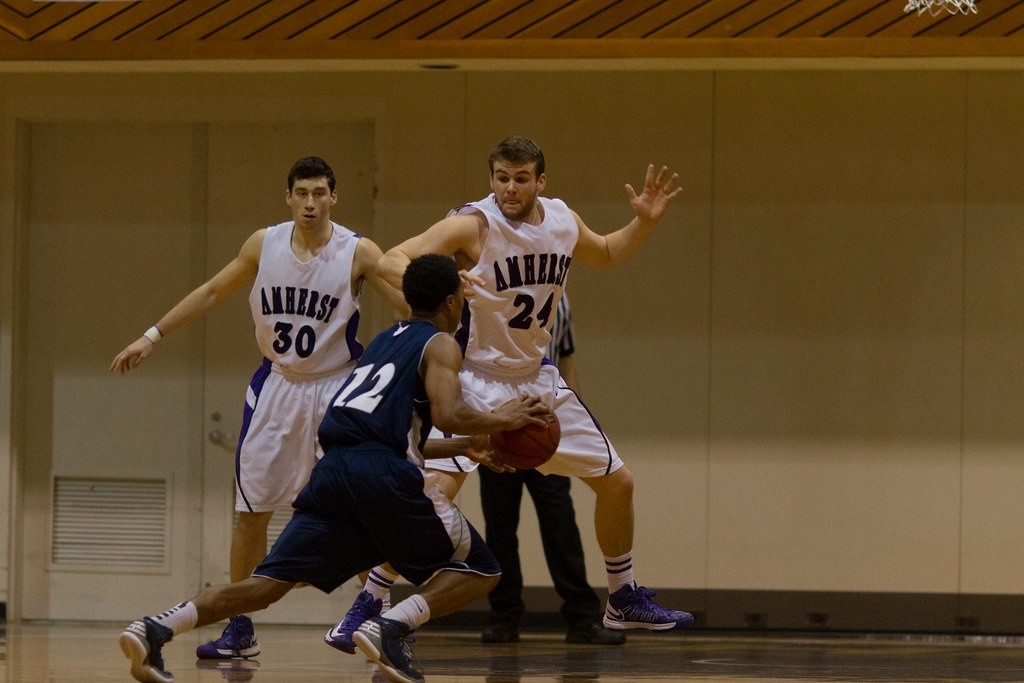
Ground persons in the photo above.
[110,135,695,658]
[120,253,550,683]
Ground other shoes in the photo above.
[563,623,627,644]
[482,622,519,642]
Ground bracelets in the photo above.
[143,324,163,344]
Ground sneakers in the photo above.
[119,616,175,683]
[352,615,425,683]
[602,582,695,632]
[324,590,383,655]
[196,613,261,660]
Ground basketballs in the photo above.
[490,397,561,469]
